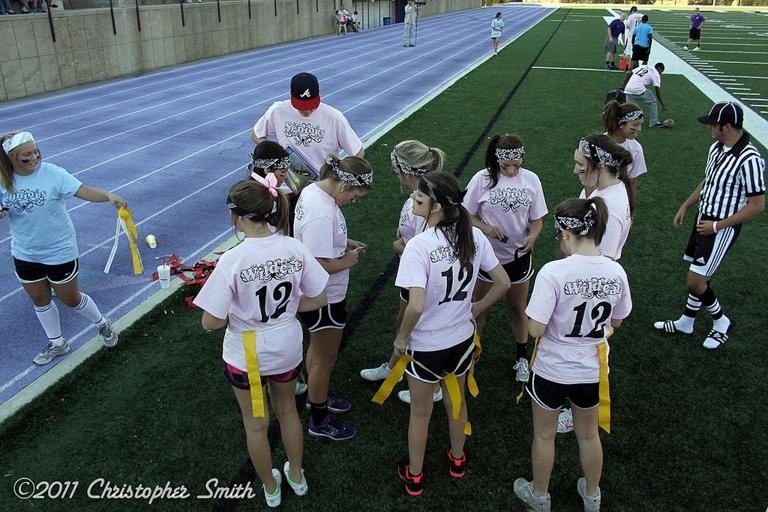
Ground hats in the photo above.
[697,101,744,126]
[290,72,320,111]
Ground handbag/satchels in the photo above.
[604,87,626,105]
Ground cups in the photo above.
[145,233,157,248]
[156,264,171,289]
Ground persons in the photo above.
[393,171,511,497]
[403,0,417,47]
[334,8,363,34]
[601,98,648,226]
[653,101,766,352]
[603,6,667,130]
[460,133,549,383]
[1,130,129,365]
[556,133,633,434]
[513,198,632,512]
[360,140,445,404]
[244,141,307,397]
[490,12,504,56]
[292,154,374,443]
[682,7,705,51]
[189,181,330,508]
[249,72,364,238]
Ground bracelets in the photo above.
[712,220,717,234]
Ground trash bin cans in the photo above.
[383,17,390,26]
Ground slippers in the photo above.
[652,320,694,335]
[703,320,736,350]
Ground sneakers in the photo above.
[603,64,620,70]
[360,364,404,382]
[98,321,119,349]
[655,123,665,128]
[682,46,689,51]
[305,392,352,413]
[693,46,701,51]
[398,387,444,403]
[32,337,71,366]
[308,414,357,440]
[295,381,308,395]
[263,468,282,508]
[513,477,551,511]
[284,460,308,495]
[514,357,530,383]
[398,456,424,496]
[446,444,466,478]
[576,476,602,511]
[556,407,574,433]
